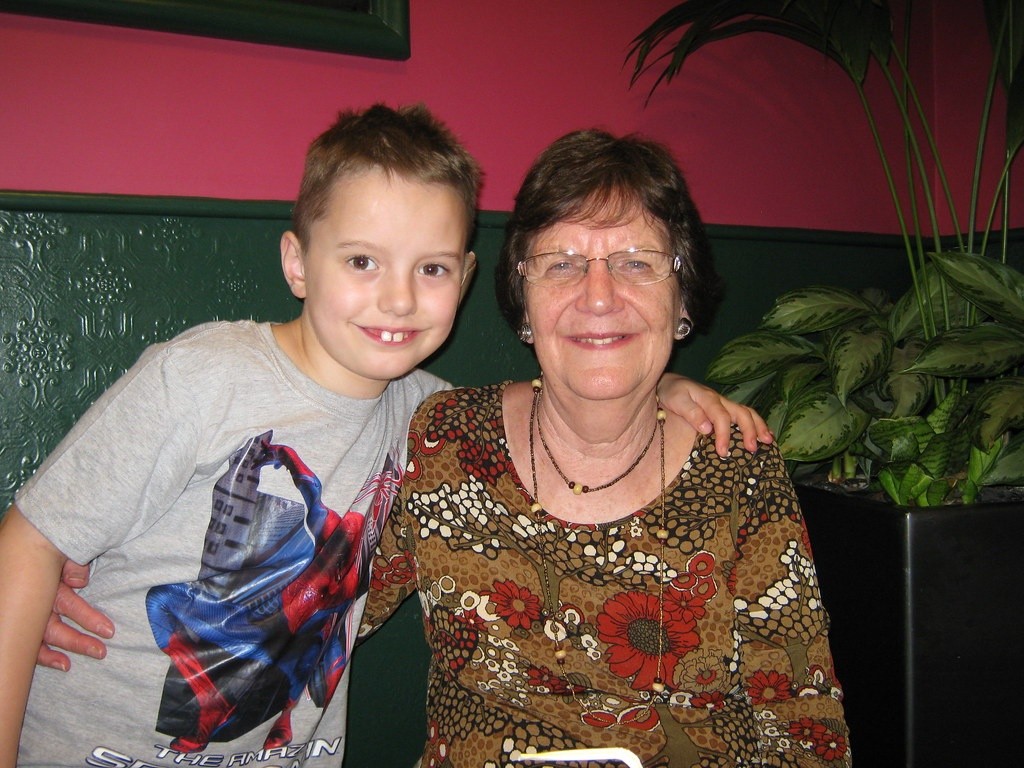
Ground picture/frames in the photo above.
[0,0,412,59]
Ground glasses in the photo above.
[517,250,682,286]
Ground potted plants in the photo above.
[621,0,1024,768]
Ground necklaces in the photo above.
[530,373,668,725]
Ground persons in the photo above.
[34,129,853,768]
[1,101,774,768]
